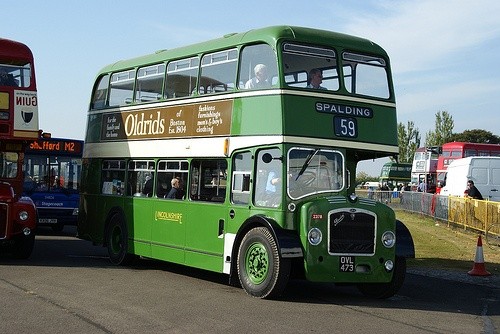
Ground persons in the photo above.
[305,69,327,90]
[464,180,483,200]
[0,69,18,88]
[142,167,316,200]
[245,63,271,89]
[48,167,55,189]
[368,173,432,204]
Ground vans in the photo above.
[444,156,500,202]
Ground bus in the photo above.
[0,38,40,254]
[435,142,500,194]
[379,162,412,192]
[20,133,85,235]
[411,146,440,193]
[84,24,414,301]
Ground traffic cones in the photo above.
[467,235,492,276]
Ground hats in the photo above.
[145,176,151,180]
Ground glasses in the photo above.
[316,75,323,78]
[467,184,473,186]
